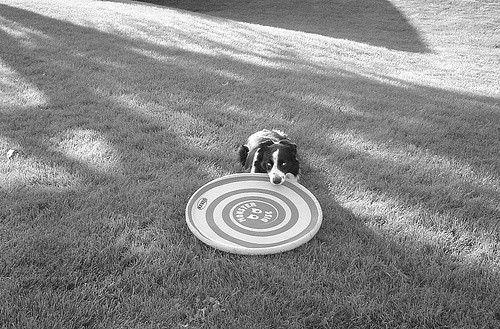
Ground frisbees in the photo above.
[185,173,323,255]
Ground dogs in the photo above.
[237,128,302,185]
